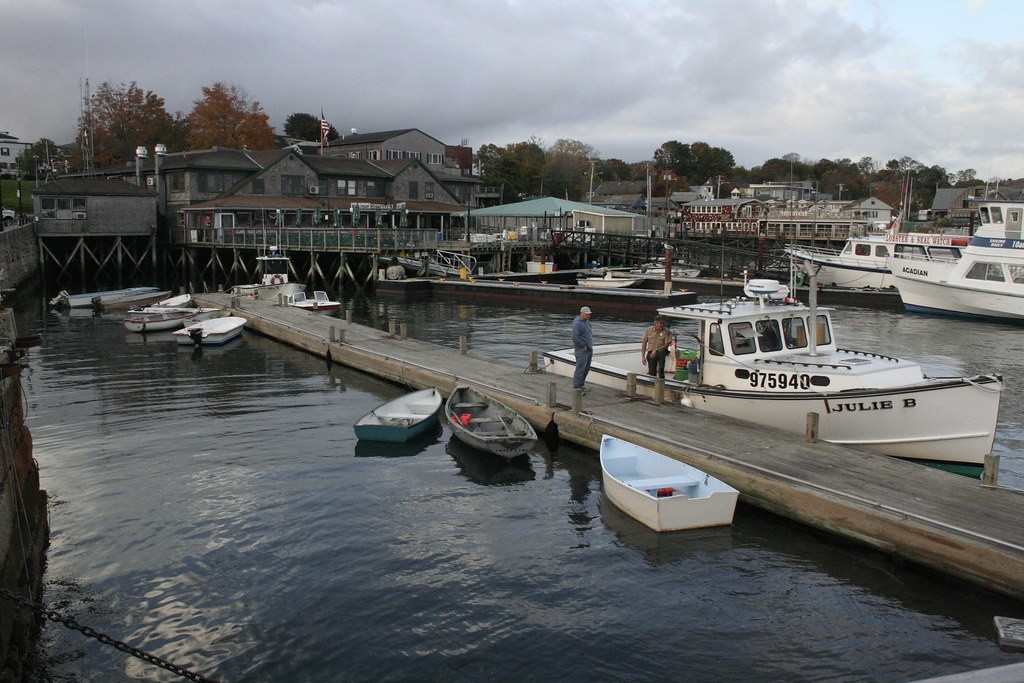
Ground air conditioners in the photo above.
[73,212,86,219]
[425,193,433,199]
[309,186,320,195]
[147,178,155,186]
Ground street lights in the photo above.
[16,174,24,224]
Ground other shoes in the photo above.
[573,388,586,396]
[580,386,591,392]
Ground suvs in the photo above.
[1,207,14,227]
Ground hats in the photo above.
[654,316,666,324]
[580,307,592,314]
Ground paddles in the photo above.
[451,409,467,430]
[495,412,516,437]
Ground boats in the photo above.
[172,316,247,344]
[48,287,160,309]
[352,384,443,443]
[143,293,191,312]
[783,207,965,290]
[630,258,702,278]
[885,197,1024,324]
[92,290,172,313]
[230,245,341,316]
[540,236,1002,467]
[124,307,223,334]
[599,433,740,533]
[576,269,645,288]
[444,386,538,460]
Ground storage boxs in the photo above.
[526,262,554,272]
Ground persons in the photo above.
[572,306,593,396]
[641,316,671,379]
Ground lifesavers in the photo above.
[271,275,284,284]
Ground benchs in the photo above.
[469,417,513,423]
[452,402,488,408]
[621,476,699,491]
[377,412,428,419]
[476,431,526,436]
